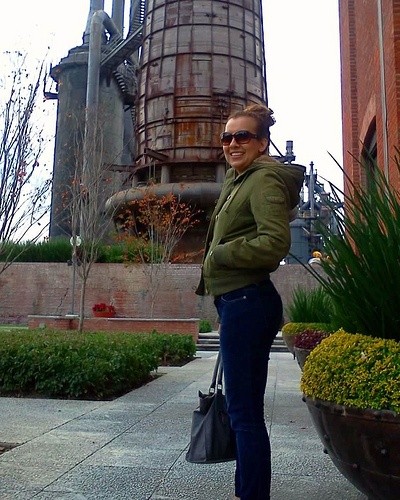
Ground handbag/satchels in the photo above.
[185,345,238,465]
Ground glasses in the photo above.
[219,130,259,146]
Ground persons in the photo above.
[195,105,306,500]
